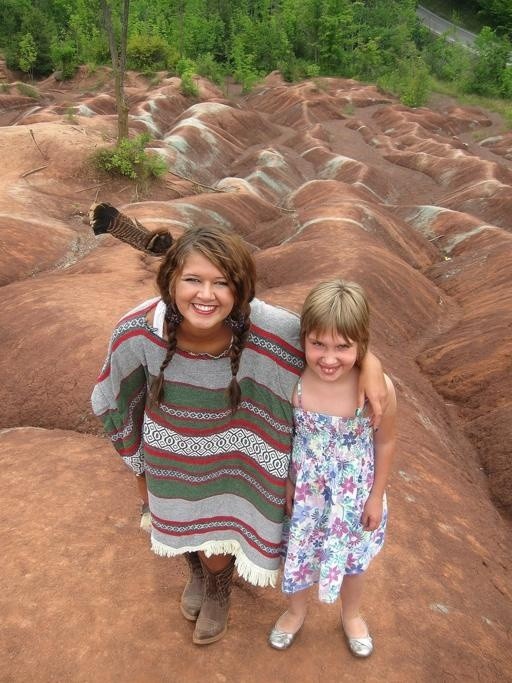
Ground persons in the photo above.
[266,279,399,657]
[87,225,390,645]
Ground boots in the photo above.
[191,553,237,644]
[178,550,207,623]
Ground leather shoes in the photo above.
[267,606,308,650]
[339,607,374,659]
